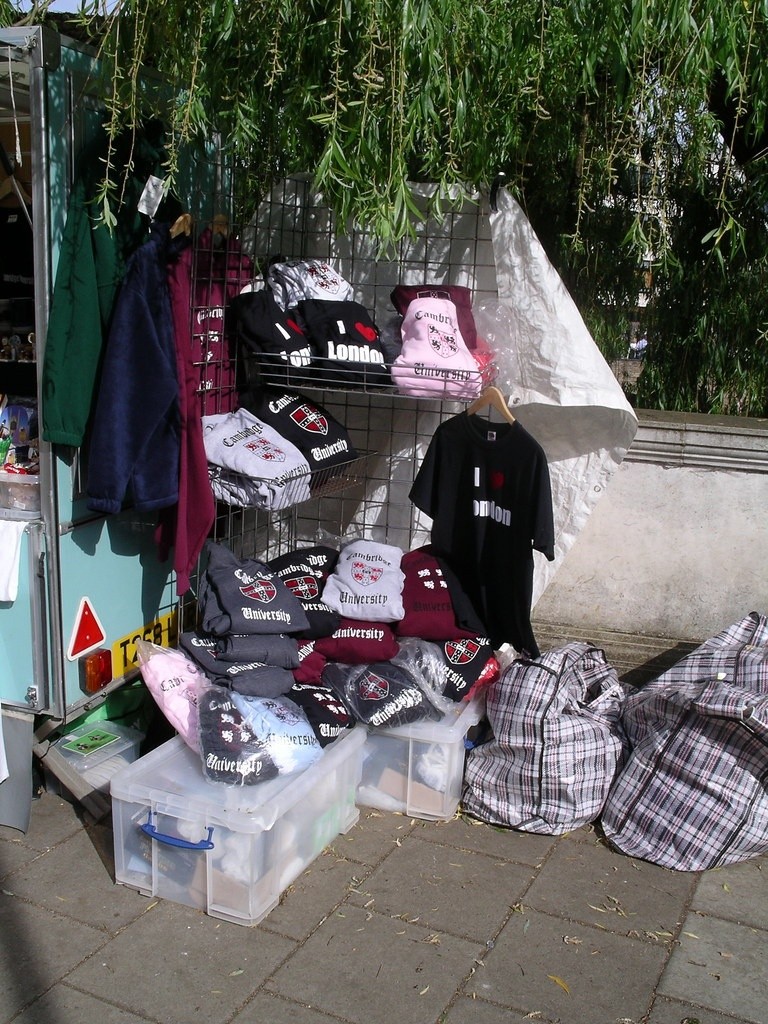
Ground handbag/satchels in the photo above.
[602,611,768,871]
[461,640,640,836]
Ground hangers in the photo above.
[467,363,515,424]
[0,155,32,205]
[208,195,231,238]
[170,193,192,238]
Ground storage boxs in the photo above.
[352,682,491,824]
[44,720,146,799]
[109,721,367,927]
[0,474,44,519]
